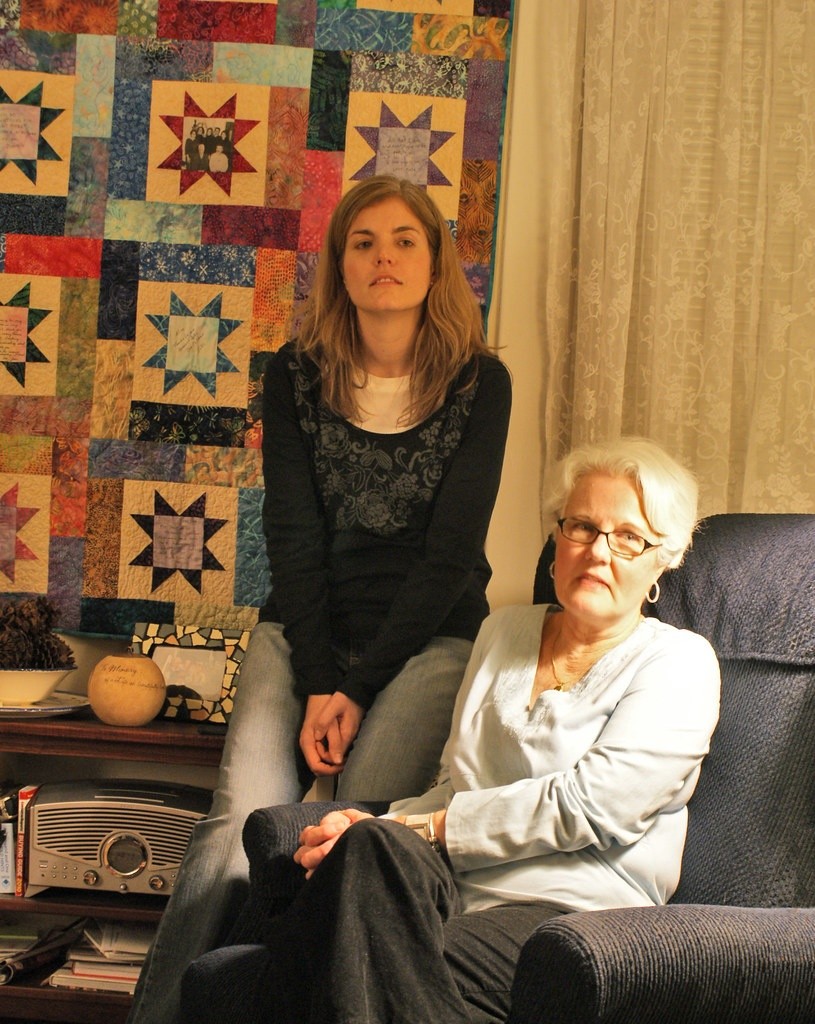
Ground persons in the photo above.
[128,173,512,1024]
[293,435,721,1024]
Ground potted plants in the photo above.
[0,596,78,706]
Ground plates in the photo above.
[0,689,92,722]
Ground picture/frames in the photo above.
[128,621,250,727]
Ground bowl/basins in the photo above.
[1,664,79,707]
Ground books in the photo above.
[0,779,160,996]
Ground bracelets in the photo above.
[404,813,440,859]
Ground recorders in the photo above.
[24,782,221,905]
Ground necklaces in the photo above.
[552,626,616,691]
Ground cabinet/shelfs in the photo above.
[0,701,339,1024]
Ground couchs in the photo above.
[241,511,815,1023]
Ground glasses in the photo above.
[556,517,660,557]
